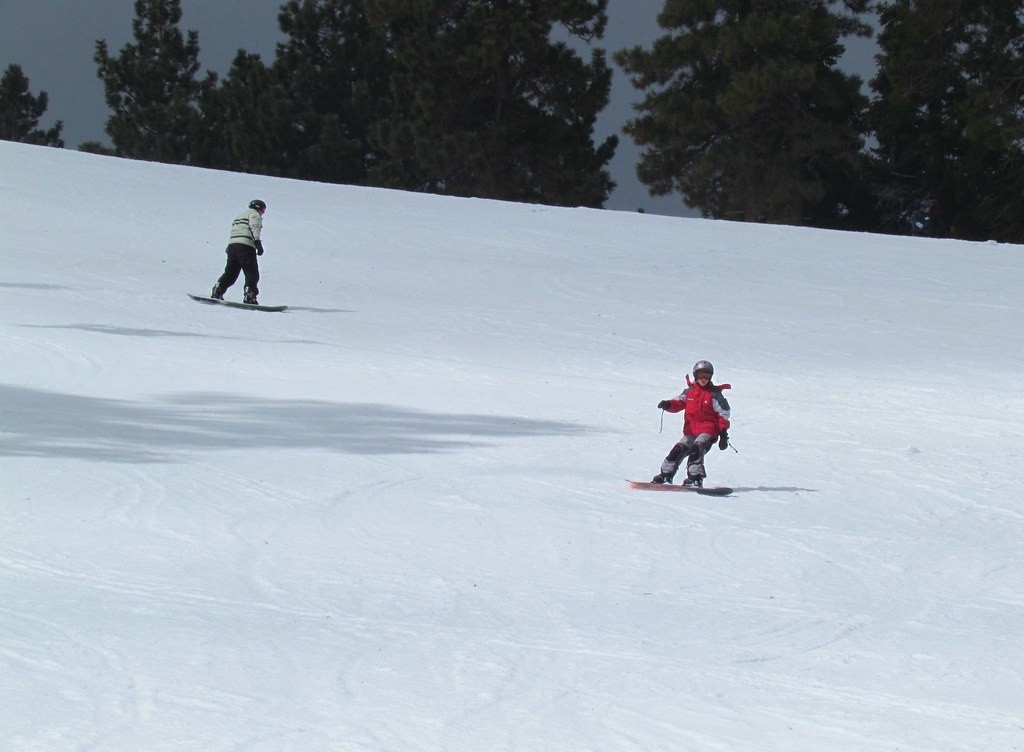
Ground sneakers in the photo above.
[653,469,679,484]
[681,473,706,488]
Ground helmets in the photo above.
[693,360,714,374]
[248,200,266,209]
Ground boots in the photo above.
[211,282,224,300]
[242,285,259,305]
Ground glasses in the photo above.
[260,207,266,214]
[695,369,712,380]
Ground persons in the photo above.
[652,361,730,488]
[212,200,266,305]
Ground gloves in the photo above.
[719,431,729,450]
[253,240,264,255]
[658,400,670,410]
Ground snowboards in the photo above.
[187,292,287,313]
[624,479,733,498]
